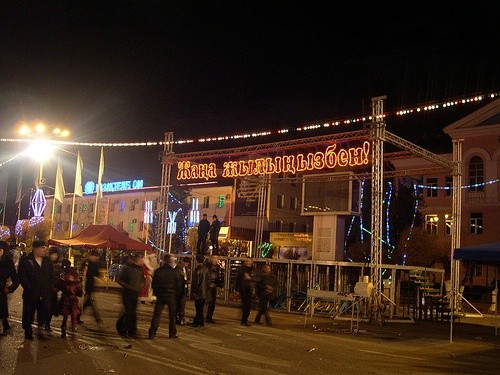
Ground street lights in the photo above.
[19,121,70,217]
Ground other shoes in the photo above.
[24,330,32,339]
[1,327,13,334]
[169,333,178,338]
[206,318,215,323]
[45,324,53,331]
[96,317,100,321]
[73,330,78,339]
[61,332,65,338]
[77,319,84,324]
[118,329,126,335]
[240,321,251,325]
[149,335,153,339]
[192,322,204,327]
[266,321,272,325]
[39,327,46,334]
[254,321,262,324]
[128,332,137,339]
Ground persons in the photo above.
[208,215,221,256]
[196,214,210,255]
[0,241,221,339]
[234,256,275,329]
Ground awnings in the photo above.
[451,241,500,266]
[46,225,153,251]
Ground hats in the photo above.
[32,240,48,247]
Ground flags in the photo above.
[55,154,65,204]
[72,150,84,198]
[98,147,105,198]
